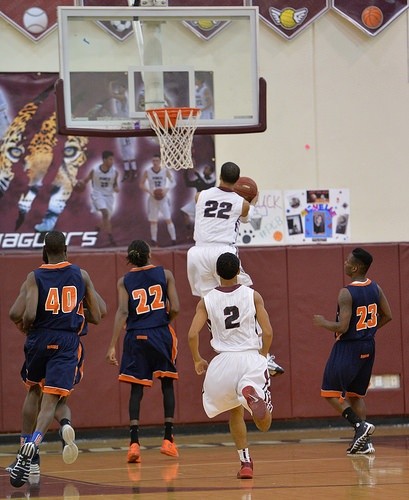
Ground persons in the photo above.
[184,161,216,242]
[138,154,182,245]
[194,75,215,120]
[186,161,284,479]
[104,80,140,182]
[314,215,324,232]
[313,247,393,454]
[74,150,120,247]
[336,216,346,234]
[110,237,183,463]
[4,225,109,492]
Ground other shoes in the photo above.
[206,319,211,331]
[7,491,26,500]
[265,353,284,377]
[172,240,176,245]
[147,239,157,247]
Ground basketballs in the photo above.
[235,177,257,202]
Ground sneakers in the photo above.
[28,448,42,473]
[29,473,40,487]
[349,421,376,454]
[59,424,78,465]
[127,443,140,462]
[242,386,272,432]
[237,457,253,478]
[346,437,375,455]
[160,440,179,457]
[352,460,376,489]
[64,484,79,500]
[241,479,253,489]
[5,448,40,473]
[128,462,141,481]
[10,442,35,487]
[24,486,40,500]
[162,463,179,482]
[347,453,375,460]
